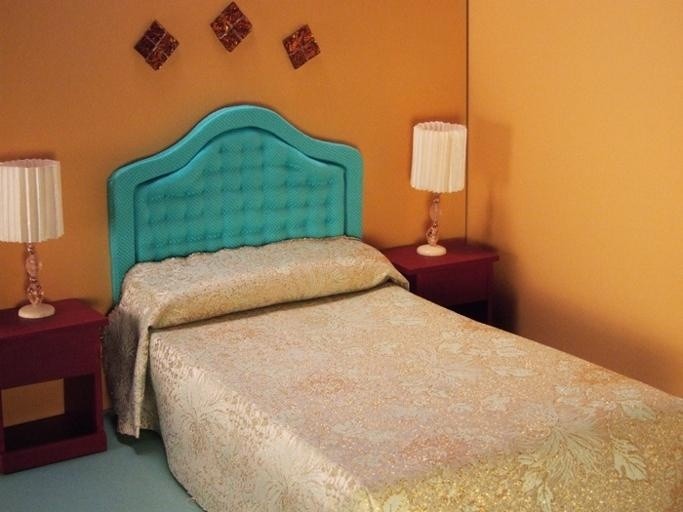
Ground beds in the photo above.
[106,101,681,511]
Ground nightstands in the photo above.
[0,298,111,474]
[379,238,498,327]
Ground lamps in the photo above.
[408,120,467,256]
[0,157,66,320]
